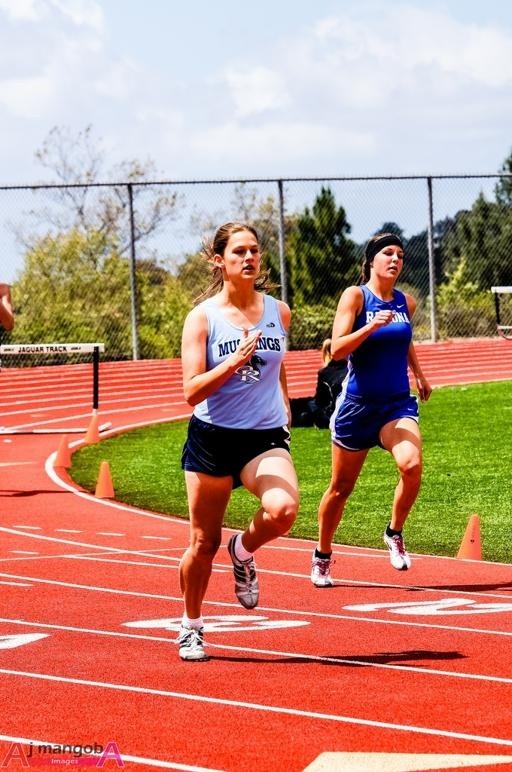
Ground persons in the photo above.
[0,282,15,333]
[175,222,301,661]
[308,233,432,588]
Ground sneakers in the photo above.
[384,522,411,571]
[178,623,209,661]
[227,533,259,609]
[311,547,334,588]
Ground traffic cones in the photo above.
[53,431,72,470]
[93,459,115,500]
[84,412,101,444]
[457,512,484,560]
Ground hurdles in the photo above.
[491,285,511,335]
[2,341,110,438]
